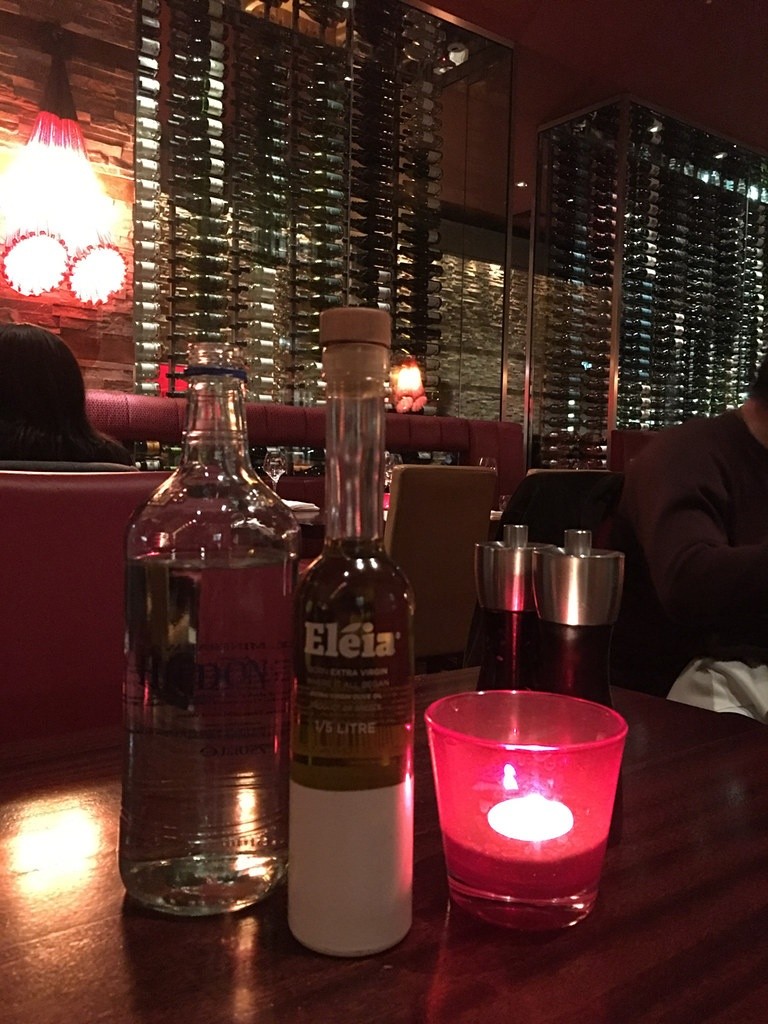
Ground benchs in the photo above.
[0,471,323,768]
[82,387,524,508]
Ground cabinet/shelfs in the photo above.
[440,252,548,434]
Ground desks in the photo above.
[0,685,768,1024]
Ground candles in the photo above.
[423,689,630,934]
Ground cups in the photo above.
[479,457,498,474]
[423,690,629,933]
[499,495,512,512]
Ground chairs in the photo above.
[596,429,668,687]
[385,463,497,659]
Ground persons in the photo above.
[0,321,135,465]
[613,353,768,693]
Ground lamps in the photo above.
[0,23,128,307]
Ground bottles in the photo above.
[524,531,625,710]
[117,339,296,919]
[130,439,460,472]
[540,109,768,469]
[134,0,442,416]
[286,307,414,957]
[473,526,536,694]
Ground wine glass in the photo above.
[384,453,403,507]
[263,451,284,492]
